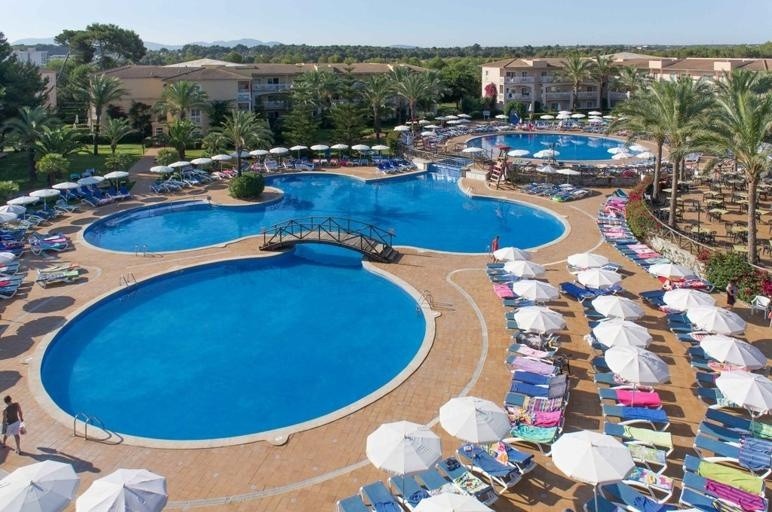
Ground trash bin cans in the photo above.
[70,168,95,182]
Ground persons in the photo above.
[492,237,499,263]
[2,395,24,457]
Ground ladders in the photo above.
[488,147,515,190]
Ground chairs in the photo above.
[596,188,675,266]
[149,155,417,194]
[415,439,537,508]
[502,404,771,512]
[486,262,752,411]
[337,474,432,510]
[522,182,591,202]
[0,179,132,299]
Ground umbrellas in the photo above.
[394,108,655,185]
[0,252,15,264]
[0,458,168,512]
